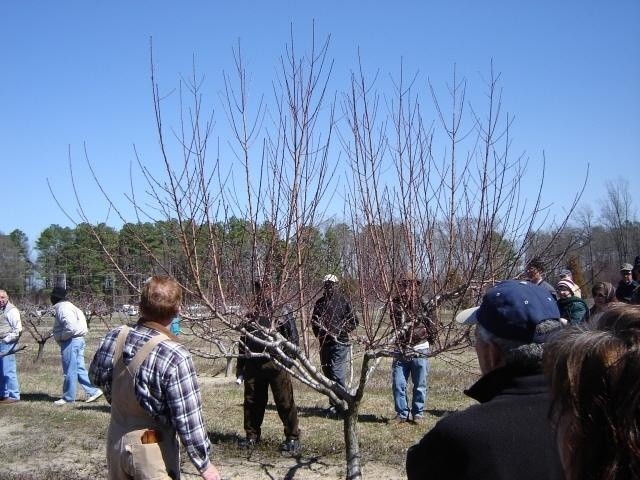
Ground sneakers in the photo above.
[412,415,424,425]
[391,412,409,424]
[85,388,103,403]
[53,398,69,406]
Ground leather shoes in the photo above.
[0,397,20,405]
[277,438,301,452]
[239,436,262,447]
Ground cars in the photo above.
[13,304,237,318]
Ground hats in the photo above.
[322,274,339,284]
[619,262,634,272]
[554,277,576,293]
[394,271,422,288]
[559,269,572,276]
[49,286,68,300]
[526,256,546,265]
[454,278,564,345]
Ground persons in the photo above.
[386,272,439,426]
[86,274,221,480]
[542,302,639,480]
[49,285,105,408]
[405,279,566,480]
[1,288,24,406]
[235,278,303,453]
[522,247,639,331]
[310,273,359,420]
